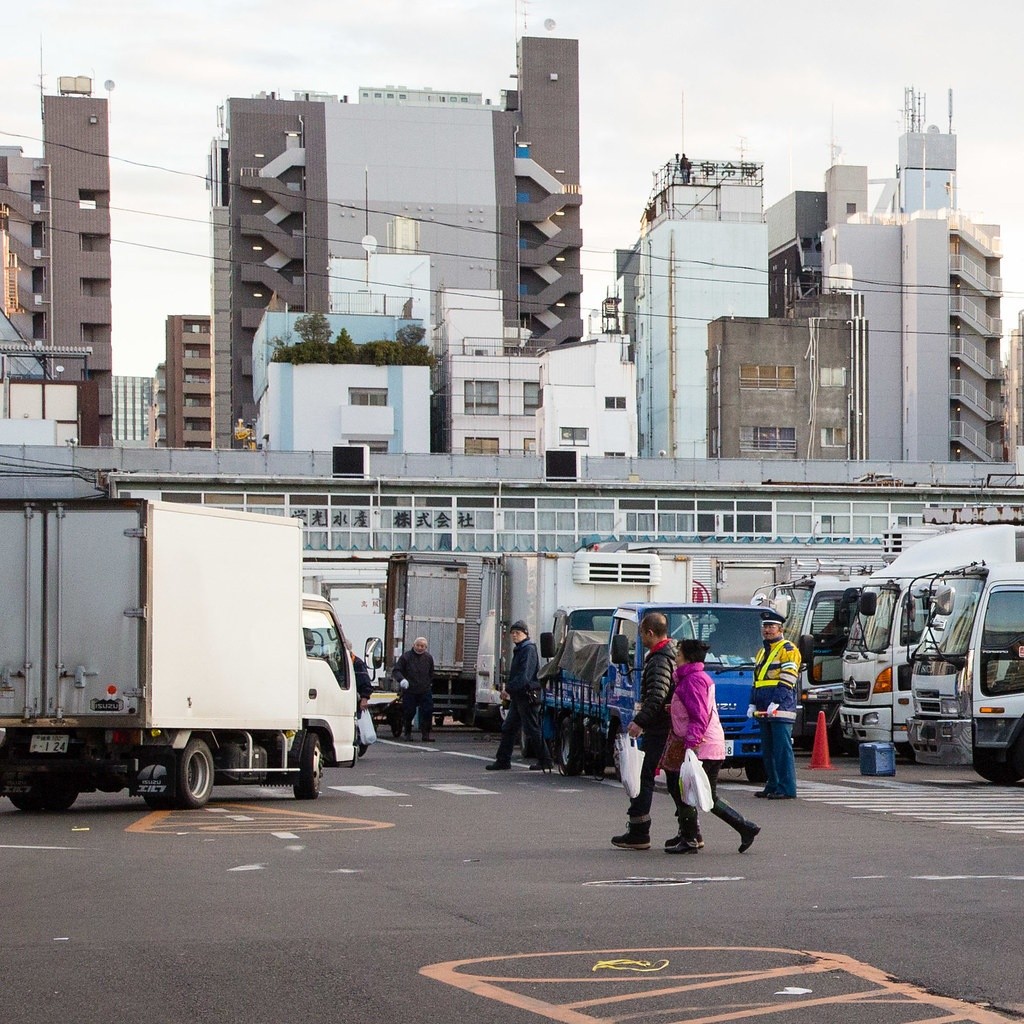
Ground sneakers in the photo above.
[530,758,555,768]
[485,760,511,769]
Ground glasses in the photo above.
[760,622,781,628]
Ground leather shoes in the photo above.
[754,788,778,796]
[766,789,795,799]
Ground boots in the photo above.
[663,815,704,853]
[403,729,413,742]
[420,729,435,742]
[710,797,761,853]
[611,814,651,849]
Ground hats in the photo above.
[760,611,786,624]
[509,619,529,636]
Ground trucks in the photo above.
[907,559,1024,786]
[537,600,787,784]
[381,549,488,725]
[476,553,692,760]
[0,495,358,810]
[748,569,883,739]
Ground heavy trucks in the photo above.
[841,526,1024,759]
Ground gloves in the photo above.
[747,703,757,718]
[767,702,779,717]
[399,678,408,688]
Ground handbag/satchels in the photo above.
[657,729,698,772]
[677,749,714,812]
[618,731,645,797]
[357,707,377,745]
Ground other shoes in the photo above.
[357,744,367,757]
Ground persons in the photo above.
[746,612,802,800]
[680,154,691,184]
[664,637,762,855]
[611,610,707,850]
[392,636,437,741]
[336,639,376,758]
[485,620,553,771]
